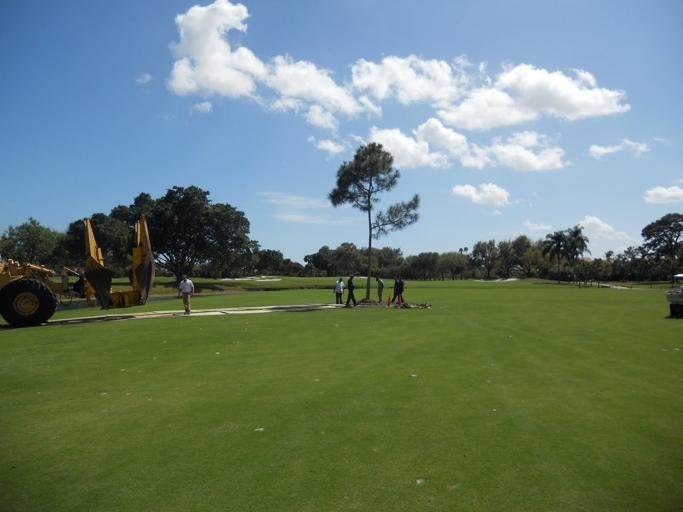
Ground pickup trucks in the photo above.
[666,273,683,316]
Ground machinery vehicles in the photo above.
[0,213,156,327]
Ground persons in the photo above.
[178,274,194,315]
[333,278,344,304]
[376,277,383,303]
[391,276,404,303]
[346,276,358,306]
